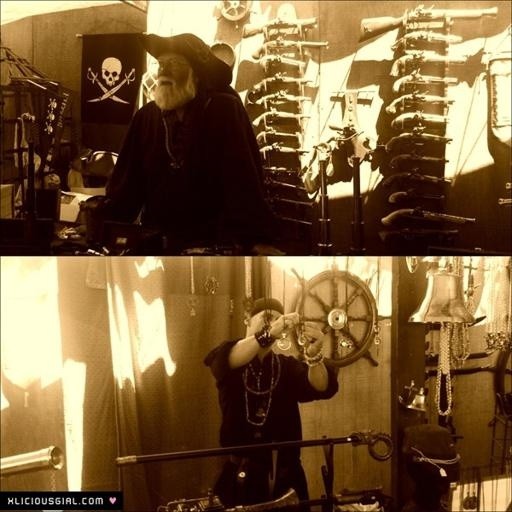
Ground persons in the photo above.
[202,295,341,512]
[69,31,289,256]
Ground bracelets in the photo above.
[303,346,324,368]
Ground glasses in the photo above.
[153,59,189,74]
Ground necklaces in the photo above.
[457,460,512,512]
[483,263,511,354]
[187,256,383,357]
[163,117,185,171]
[433,321,472,417]
[242,349,283,427]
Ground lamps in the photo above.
[408,275,474,424]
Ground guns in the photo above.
[240,17,328,227]
[357,6,498,226]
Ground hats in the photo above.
[142,34,232,89]
[250,298,284,319]
